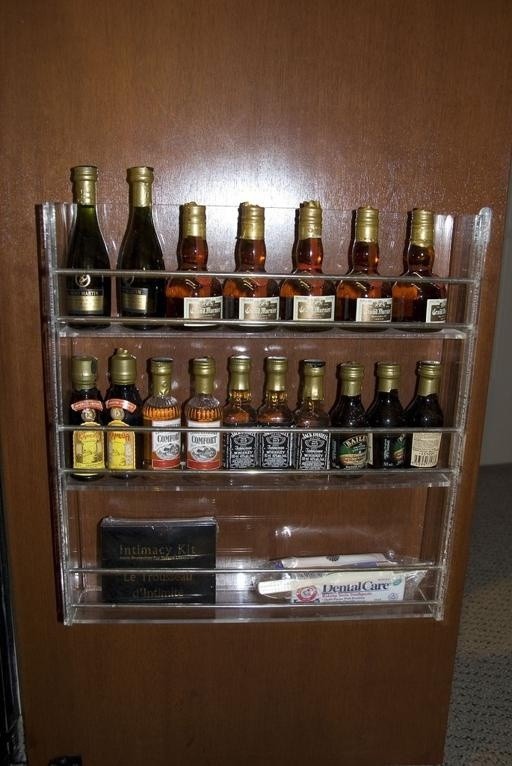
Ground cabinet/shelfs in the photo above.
[43,201,493,629]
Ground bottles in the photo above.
[327,360,368,480]
[181,356,223,479]
[336,207,393,331]
[223,203,280,332]
[143,357,182,480]
[393,209,449,331]
[104,348,143,479]
[294,359,332,480]
[68,354,105,481]
[280,201,335,331]
[366,361,407,470]
[166,201,223,331]
[404,360,445,470]
[116,167,166,330]
[222,354,258,470]
[61,164,113,330]
[258,356,294,469]
